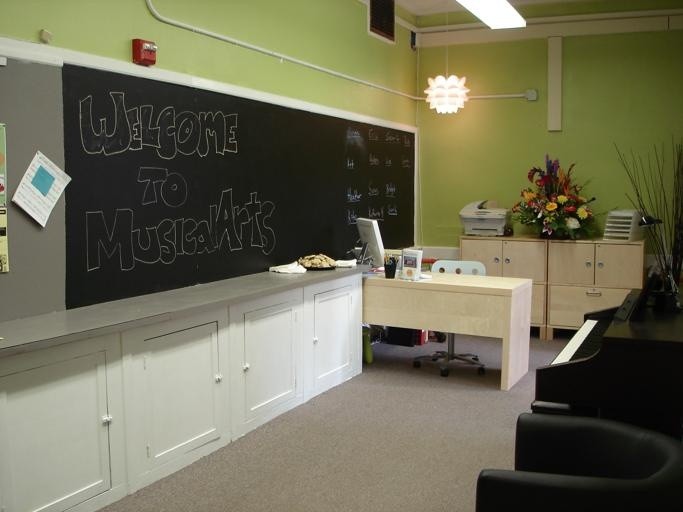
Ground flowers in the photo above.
[508,154,597,241]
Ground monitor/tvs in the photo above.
[356,218,384,269]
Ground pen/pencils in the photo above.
[383,251,399,266]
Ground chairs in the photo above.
[474,411,682,512]
[413,260,486,377]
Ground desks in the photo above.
[362,266,533,391]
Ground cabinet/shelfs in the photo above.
[0,334,125,512]
[308,272,362,397]
[125,287,305,499]
[545,238,646,343]
[458,235,547,341]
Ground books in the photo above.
[397,247,422,280]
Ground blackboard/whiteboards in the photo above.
[63,64,419,312]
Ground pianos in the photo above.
[531,288,681,440]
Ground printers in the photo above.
[458,200,510,237]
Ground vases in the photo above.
[541,227,570,241]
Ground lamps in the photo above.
[422,1,470,116]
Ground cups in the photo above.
[383,263,396,278]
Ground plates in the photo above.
[301,266,336,272]
